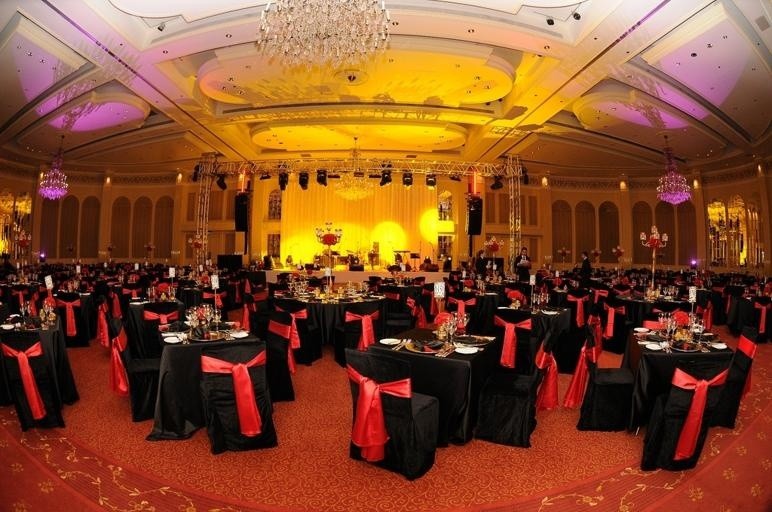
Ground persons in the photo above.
[576,251,592,287]
[475,250,492,278]
[513,247,532,284]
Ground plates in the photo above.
[380,312,488,357]
[633,311,728,354]
[157,319,250,343]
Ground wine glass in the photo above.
[286,270,382,303]
[527,290,558,317]
[1,299,59,332]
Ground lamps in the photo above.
[38,136,69,201]
[641,227,668,290]
[558,246,625,271]
[483,236,504,276]
[254,0,391,74]
[190,239,202,276]
[657,136,693,205]
[316,222,343,275]
[18,230,31,267]
